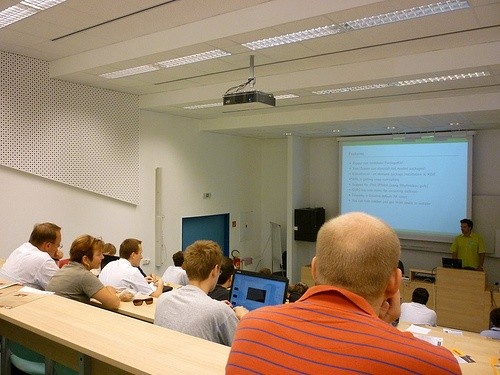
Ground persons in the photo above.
[162,251,189,286]
[449,218,487,271]
[90,243,116,276]
[226,211,463,375]
[480,308,500,340]
[210,257,235,300]
[289,283,308,302]
[157,240,249,346]
[0,222,64,290]
[400,288,437,327]
[99,239,163,297]
[46,234,132,310]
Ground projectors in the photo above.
[223,90,275,108]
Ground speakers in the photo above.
[294,207,326,242]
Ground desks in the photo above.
[0,279,500,375]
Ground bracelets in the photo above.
[481,266,483,268]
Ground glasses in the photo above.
[133,297,153,306]
[46,240,63,249]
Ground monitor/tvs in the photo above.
[229,269,289,311]
[442,258,462,270]
